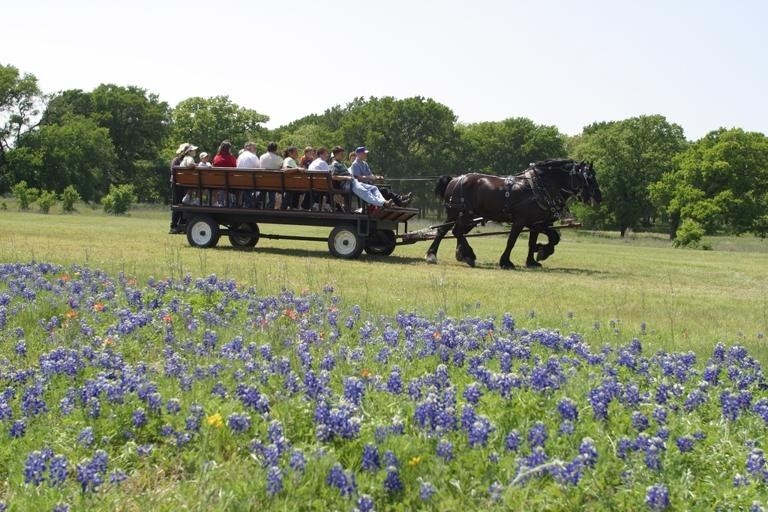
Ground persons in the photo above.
[170,139,355,213]
[330,146,394,208]
[349,147,412,207]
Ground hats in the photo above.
[199,152,208,159]
[356,147,370,153]
[176,143,199,158]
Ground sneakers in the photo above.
[385,199,395,208]
[182,197,201,206]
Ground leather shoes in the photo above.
[401,192,413,208]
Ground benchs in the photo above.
[172,166,372,211]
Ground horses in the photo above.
[425,158,592,264]
[454,158,602,263]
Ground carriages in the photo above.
[167,146,603,274]
